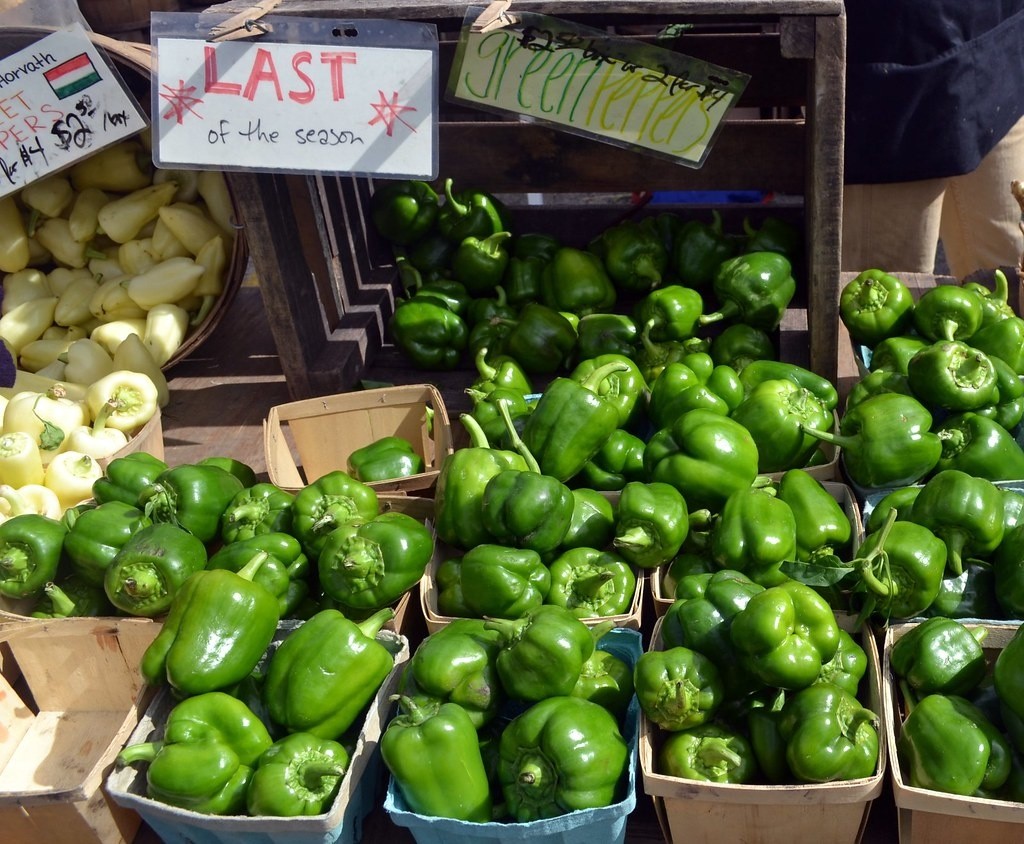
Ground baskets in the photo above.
[1,25,247,377]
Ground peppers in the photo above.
[0,127,1024,824]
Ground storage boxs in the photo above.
[0,276,1024,844]
